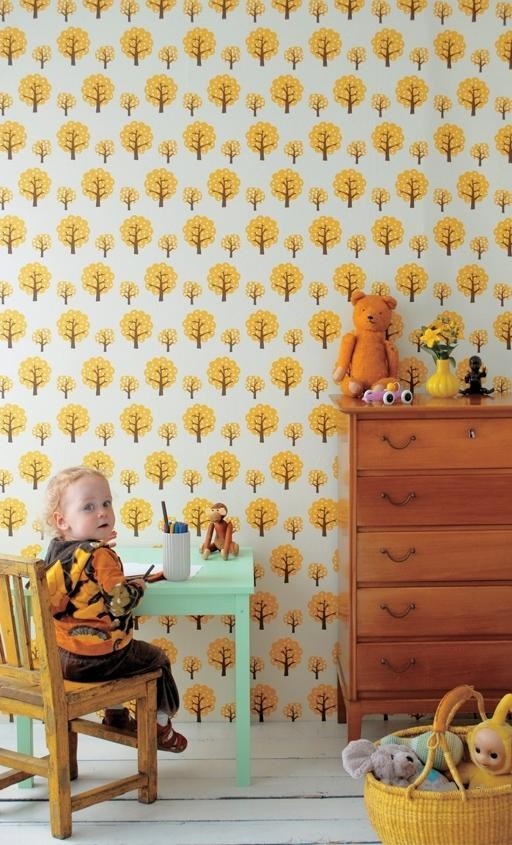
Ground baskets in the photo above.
[364,684,512,845]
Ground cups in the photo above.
[161,531,189,582]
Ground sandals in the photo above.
[156,718,188,753]
[104,707,137,733]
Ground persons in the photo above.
[447,692,512,791]
[457,355,494,396]
[24,464,189,753]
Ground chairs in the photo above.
[2,558,164,838]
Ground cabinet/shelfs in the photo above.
[330,394,510,745]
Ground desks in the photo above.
[1,544,257,797]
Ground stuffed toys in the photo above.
[340,737,457,793]
[332,288,400,398]
[381,730,465,770]
[199,501,239,561]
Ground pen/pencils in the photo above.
[142,566,157,580]
[158,502,189,533]
